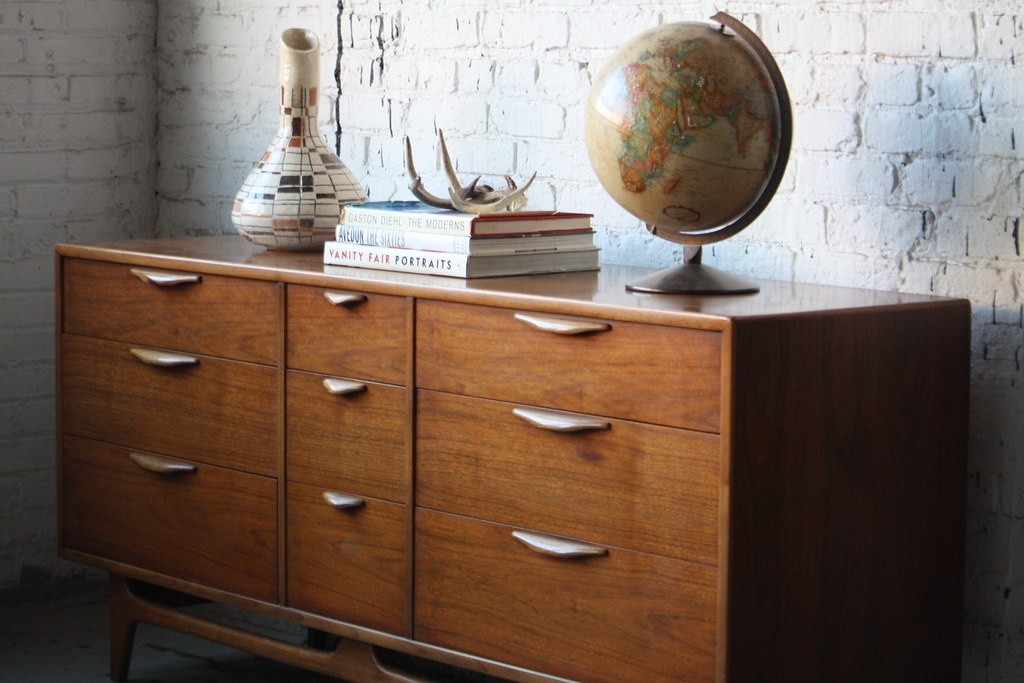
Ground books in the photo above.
[322,200,601,279]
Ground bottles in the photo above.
[230,27,373,254]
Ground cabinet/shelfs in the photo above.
[56,232,972,683]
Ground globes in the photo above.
[580,10,795,296]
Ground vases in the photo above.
[231,28,368,251]
